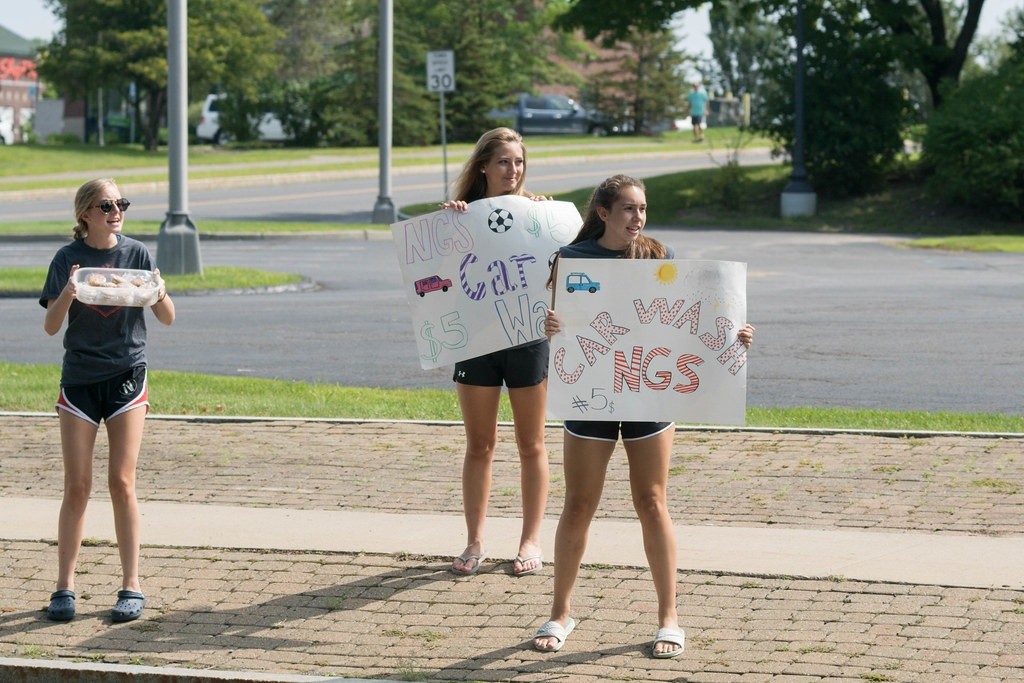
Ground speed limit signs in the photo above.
[424,48,455,93]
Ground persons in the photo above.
[441,128,554,575]
[534,176,687,654]
[38,176,174,621]
[681,78,713,145]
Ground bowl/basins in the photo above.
[72,267,161,307]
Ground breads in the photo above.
[86,273,155,305]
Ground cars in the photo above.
[196,93,315,147]
[641,110,708,132]
[481,91,631,138]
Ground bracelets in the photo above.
[157,290,167,303]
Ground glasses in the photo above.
[87,198,131,214]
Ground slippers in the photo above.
[111,590,145,622]
[652,626,684,658]
[47,590,77,621]
[514,546,543,577]
[452,552,488,575]
[534,616,575,652]
